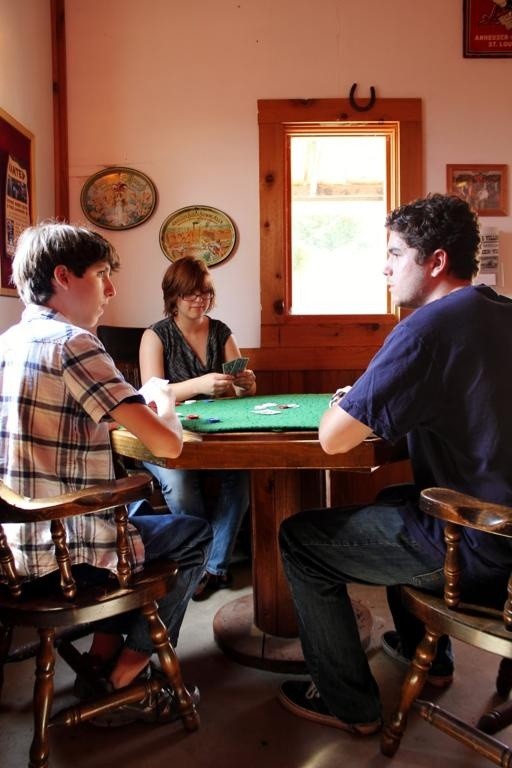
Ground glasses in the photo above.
[178,290,213,301]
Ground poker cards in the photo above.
[137,356,299,415]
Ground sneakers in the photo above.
[191,570,233,600]
[73,650,200,727]
[381,629,454,688]
[277,680,383,735]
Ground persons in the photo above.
[273,192,512,736]
[138,254,257,602]
[1,222,201,730]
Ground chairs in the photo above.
[378,487,512,768]
[114,365,168,514]
[0,477,199,768]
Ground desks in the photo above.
[111,392,408,673]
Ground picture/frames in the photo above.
[463,0,512,58]
[446,163,508,217]
[0,107,36,297]
